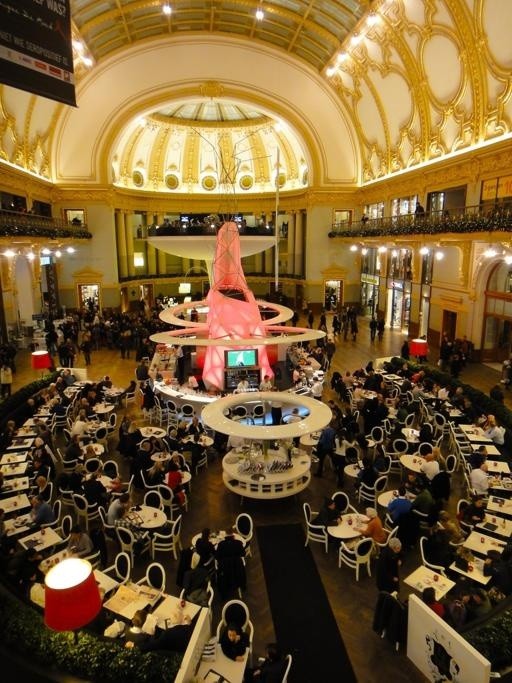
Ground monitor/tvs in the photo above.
[224,349,258,369]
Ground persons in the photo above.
[2,199,40,215]
[41,302,198,368]
[289,293,386,342]
[361,213,369,224]
[415,202,426,223]
[287,331,509,682]
[1,359,288,681]
